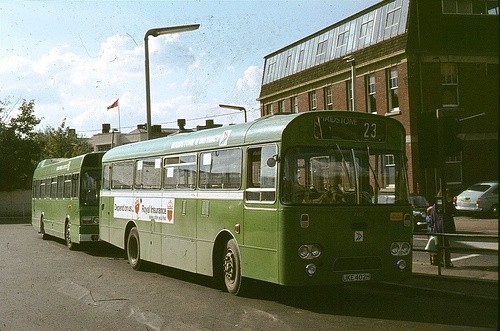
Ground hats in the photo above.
[434,192,443,197]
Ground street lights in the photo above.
[219,104,247,122]
[144,24,200,139]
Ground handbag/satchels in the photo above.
[425,236,438,251]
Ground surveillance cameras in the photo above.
[343,55,355,63]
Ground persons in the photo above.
[431,188,456,269]
[426,206,435,233]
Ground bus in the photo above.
[98,109,413,295]
[32,151,107,250]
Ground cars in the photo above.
[453,183,499,219]
[371,194,432,233]
